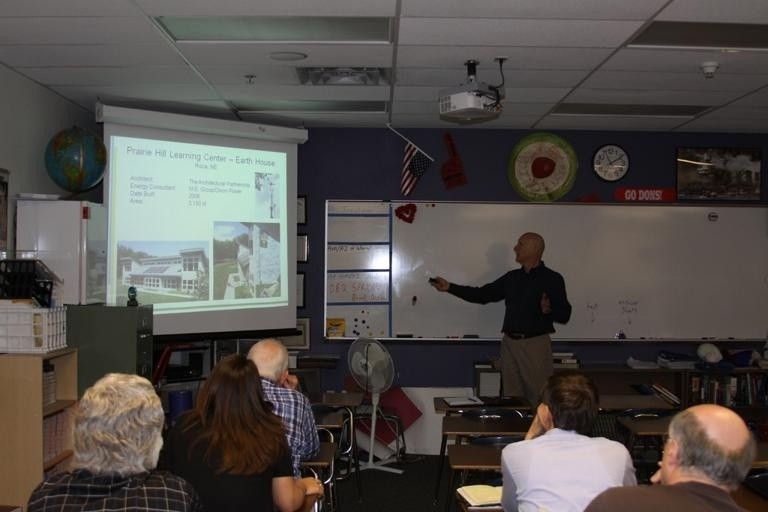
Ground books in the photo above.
[657,350,697,370]
[553,352,578,368]
[456,484,503,506]
[43,372,58,407]
[649,382,680,404]
[690,375,768,403]
[44,411,69,461]
[475,360,494,369]
[443,395,483,408]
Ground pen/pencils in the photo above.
[468,397,477,402]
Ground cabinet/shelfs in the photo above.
[0,347,78,508]
[149,340,323,438]
[684,363,768,440]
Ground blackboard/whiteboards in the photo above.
[324,200,767,342]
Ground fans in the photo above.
[340,335,405,474]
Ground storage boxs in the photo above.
[0,305,68,355]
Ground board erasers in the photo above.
[397,333,414,337]
[464,334,478,339]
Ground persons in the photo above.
[582,403,768,512]
[429,233,573,404]
[165,354,324,512]
[502,369,639,512]
[246,339,321,479]
[26,371,197,512]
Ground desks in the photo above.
[432,395,676,416]
[432,413,533,508]
[474,357,681,396]
[616,414,671,452]
[445,444,504,509]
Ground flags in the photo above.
[400,142,435,197]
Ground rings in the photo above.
[320,483,322,487]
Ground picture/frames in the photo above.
[297,272,306,311]
[297,234,309,264]
[297,196,308,226]
[675,144,763,204]
[276,317,311,350]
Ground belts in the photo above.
[503,330,547,340]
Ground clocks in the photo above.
[592,144,630,184]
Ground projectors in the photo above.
[438,90,504,125]
